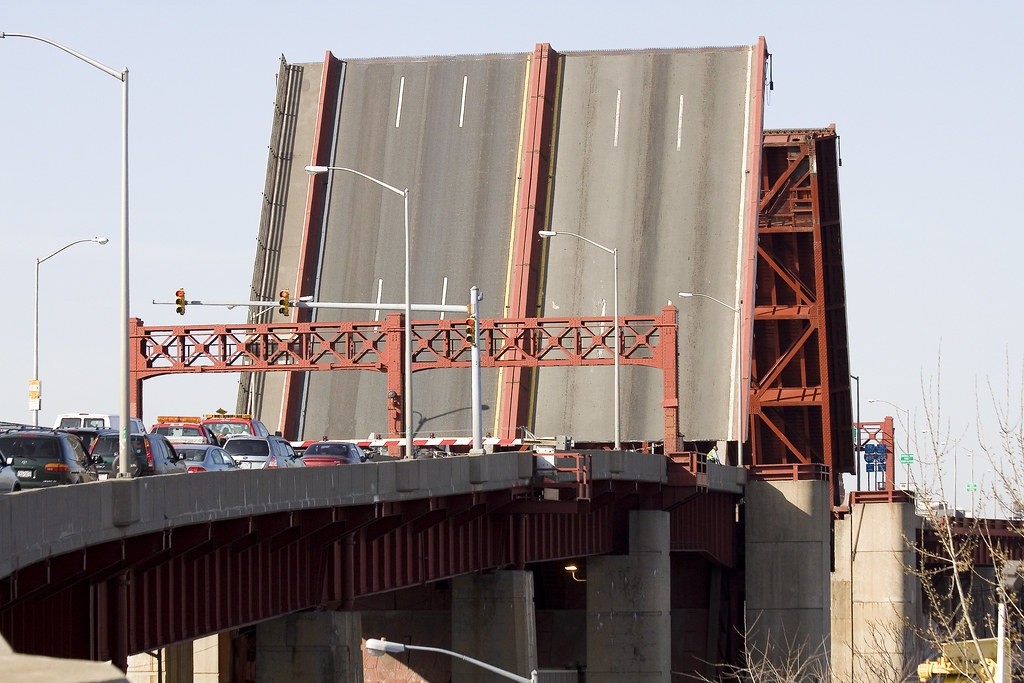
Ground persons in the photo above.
[706,446,720,465]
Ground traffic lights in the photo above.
[175,287,185,315]
[278,288,291,316]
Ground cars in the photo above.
[1,404,304,432]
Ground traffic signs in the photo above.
[862,442,886,473]
[901,453,913,464]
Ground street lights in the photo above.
[868,397,912,488]
[305,160,419,464]
[679,291,746,469]
[540,227,625,452]
[33,236,111,430]
[921,428,958,517]
